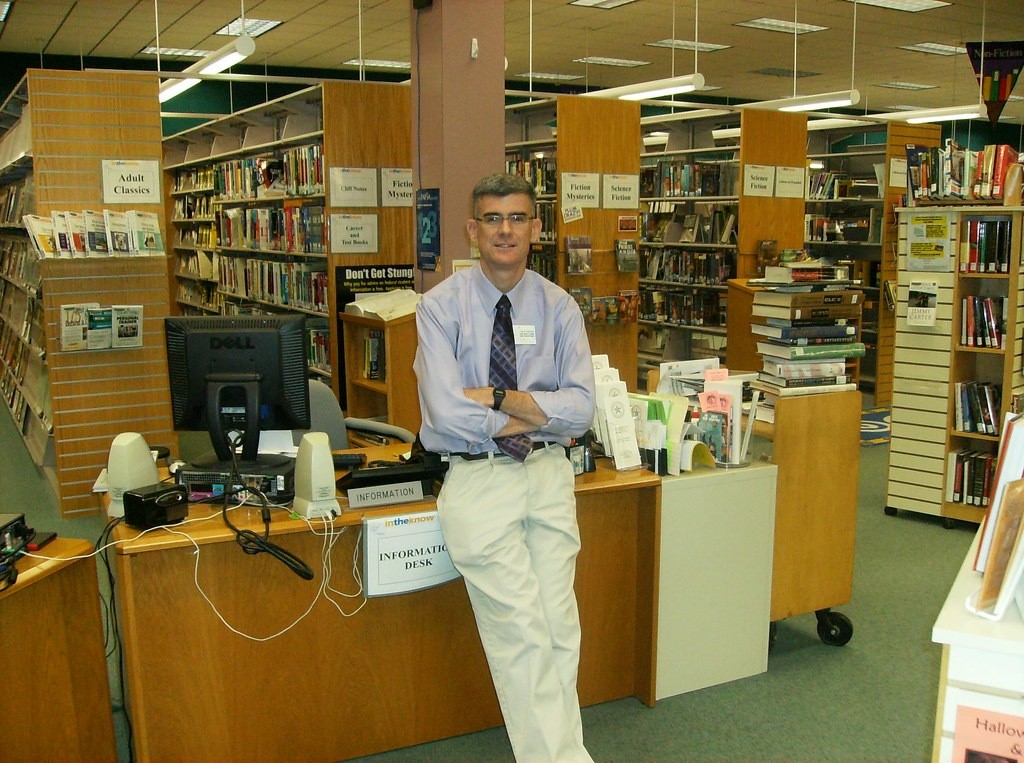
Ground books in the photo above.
[639,284,728,329]
[0,183,53,433]
[960,295,1008,350]
[507,159,556,284]
[960,220,1011,274]
[945,447,998,508]
[654,161,720,197]
[641,248,735,289]
[905,138,1024,203]
[954,380,999,437]
[60,303,144,352]
[172,165,220,311]
[805,214,834,240]
[810,170,850,200]
[746,263,868,424]
[364,329,386,383]
[221,142,330,318]
[22,209,166,260]
[305,328,332,372]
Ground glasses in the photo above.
[474,214,534,225]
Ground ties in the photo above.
[490,296,532,463]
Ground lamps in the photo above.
[357,0,986,156]
[155,0,257,104]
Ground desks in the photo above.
[104,441,661,763]
[0,537,120,763]
[660,460,777,702]
[932,516,1023,763]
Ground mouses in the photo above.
[168,461,185,476]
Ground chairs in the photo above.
[291,379,417,450]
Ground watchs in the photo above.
[491,386,507,409]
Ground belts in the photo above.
[451,440,557,462]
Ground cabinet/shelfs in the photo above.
[646,365,862,646]
[886,207,1024,529]
[0,67,942,520]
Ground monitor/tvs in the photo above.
[165,315,312,470]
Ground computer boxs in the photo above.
[0,512,29,570]
[176,455,300,506]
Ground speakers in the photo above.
[292,432,342,518]
[108,432,160,517]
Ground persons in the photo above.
[413,174,596,763]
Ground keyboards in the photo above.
[332,453,368,469]
